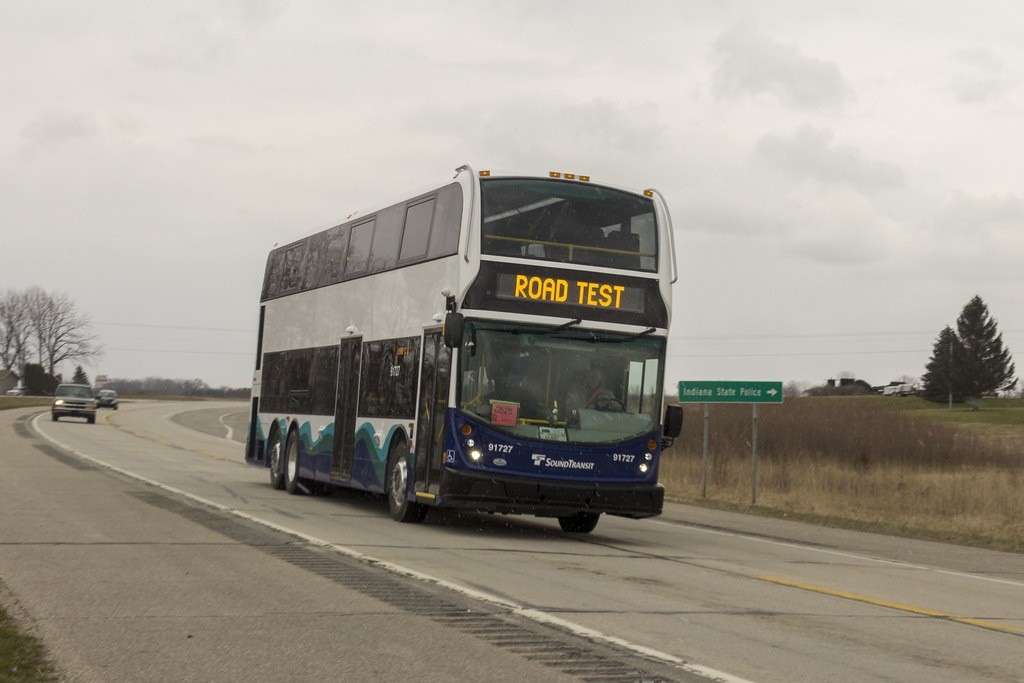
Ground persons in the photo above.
[564,366,622,419]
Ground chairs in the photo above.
[544,244,570,262]
[606,231,642,270]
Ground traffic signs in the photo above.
[676,380,784,405]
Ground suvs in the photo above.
[50,382,97,425]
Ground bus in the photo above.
[242,163,684,536]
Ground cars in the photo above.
[6,386,24,395]
[96,389,120,411]
